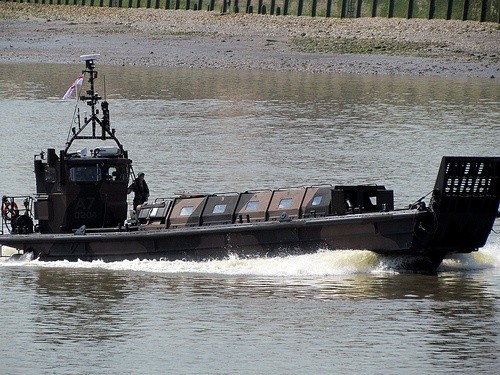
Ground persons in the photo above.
[127,172,149,210]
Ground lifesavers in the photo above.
[3,200,18,220]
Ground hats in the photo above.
[137,172,145,177]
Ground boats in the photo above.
[0,54,500,273]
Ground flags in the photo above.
[62,74,84,99]
